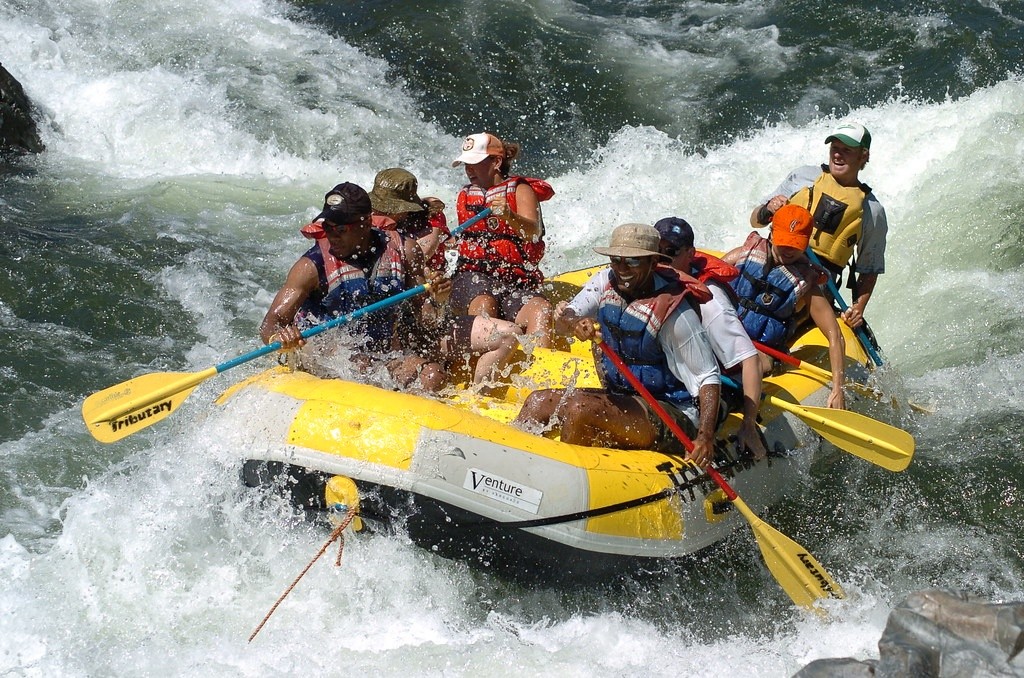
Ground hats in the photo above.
[592,223,675,264]
[771,204,814,251]
[654,217,694,248]
[368,168,427,214]
[824,121,871,151]
[312,181,372,225]
[451,131,503,168]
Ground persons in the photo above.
[514,121,889,470]
[258,129,555,395]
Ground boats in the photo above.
[209,248,873,596]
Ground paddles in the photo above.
[581,243,935,622]
[448,206,494,236]
[82,284,426,444]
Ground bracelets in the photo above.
[429,296,445,308]
[269,334,284,353]
[503,203,511,219]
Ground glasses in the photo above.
[610,256,650,267]
[658,242,687,257]
[322,221,357,235]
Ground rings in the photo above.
[704,458,708,462]
[583,326,587,331]
